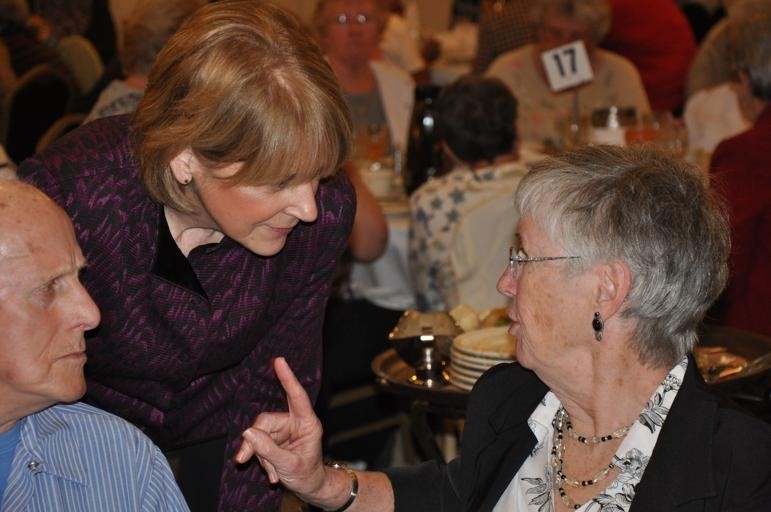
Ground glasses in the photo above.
[504,243,600,284]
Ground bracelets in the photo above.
[327,451,359,511]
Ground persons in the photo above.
[236,140,771,511]
[2,164,192,511]
[15,2,360,510]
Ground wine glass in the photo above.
[386,335,452,388]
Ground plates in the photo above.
[446,326,518,392]
[369,326,771,410]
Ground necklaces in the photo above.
[542,398,634,508]
